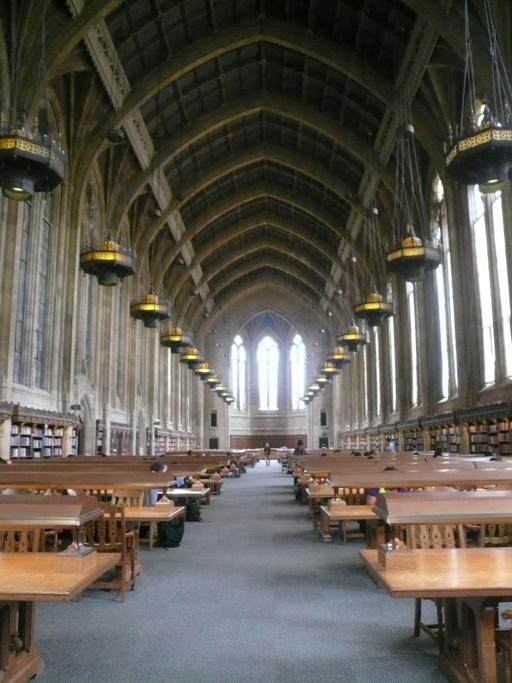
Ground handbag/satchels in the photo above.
[186,499,202,521]
[154,518,184,547]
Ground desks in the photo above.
[0,446,275,683]
[277,447,511,683]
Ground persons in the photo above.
[293,439,307,454]
[366,465,412,505]
[41,488,77,550]
[280,443,287,450]
[264,441,271,467]
[145,462,168,504]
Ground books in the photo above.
[338,412,512,454]
[10,420,198,458]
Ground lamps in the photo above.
[387,106,444,277]
[131,222,172,328]
[354,199,392,327]
[1,0,70,199]
[178,256,237,407]
[81,137,139,288]
[441,1,510,183]
[159,289,187,354]
[299,229,353,406]
